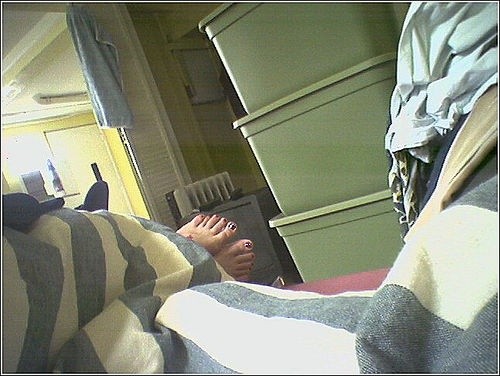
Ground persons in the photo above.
[3,212,254,375]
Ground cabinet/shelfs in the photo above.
[179,187,303,288]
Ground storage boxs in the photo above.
[268,188,408,283]
[199,3,411,114]
[232,52,399,217]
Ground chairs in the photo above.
[74,181,109,212]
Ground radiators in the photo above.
[173,172,235,218]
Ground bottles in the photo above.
[46,159,66,198]
